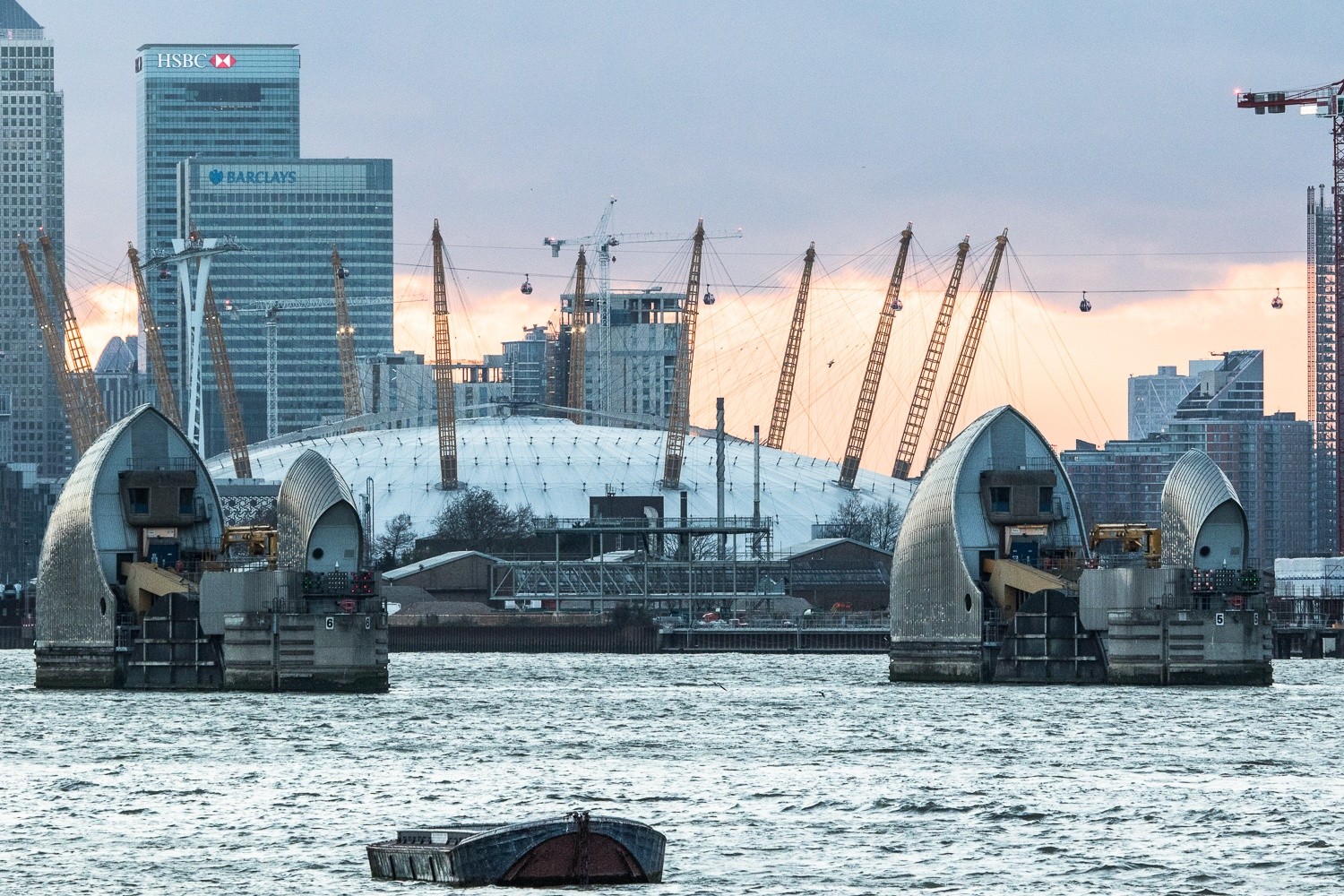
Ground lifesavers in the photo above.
[340,599,355,611]
[1230,595,1244,607]
[1089,558,1097,568]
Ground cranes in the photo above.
[1234,77,1344,557]
[543,195,743,422]
